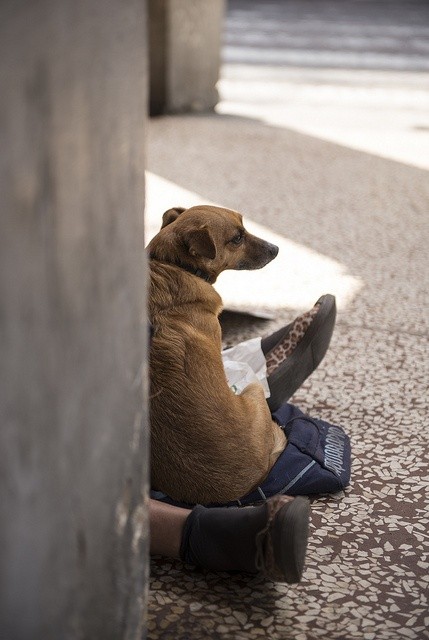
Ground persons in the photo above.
[148,294,337,586]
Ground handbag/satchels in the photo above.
[150,404,350,509]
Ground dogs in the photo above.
[146,203,288,512]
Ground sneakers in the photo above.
[180,495,308,584]
[222,295,335,414]
